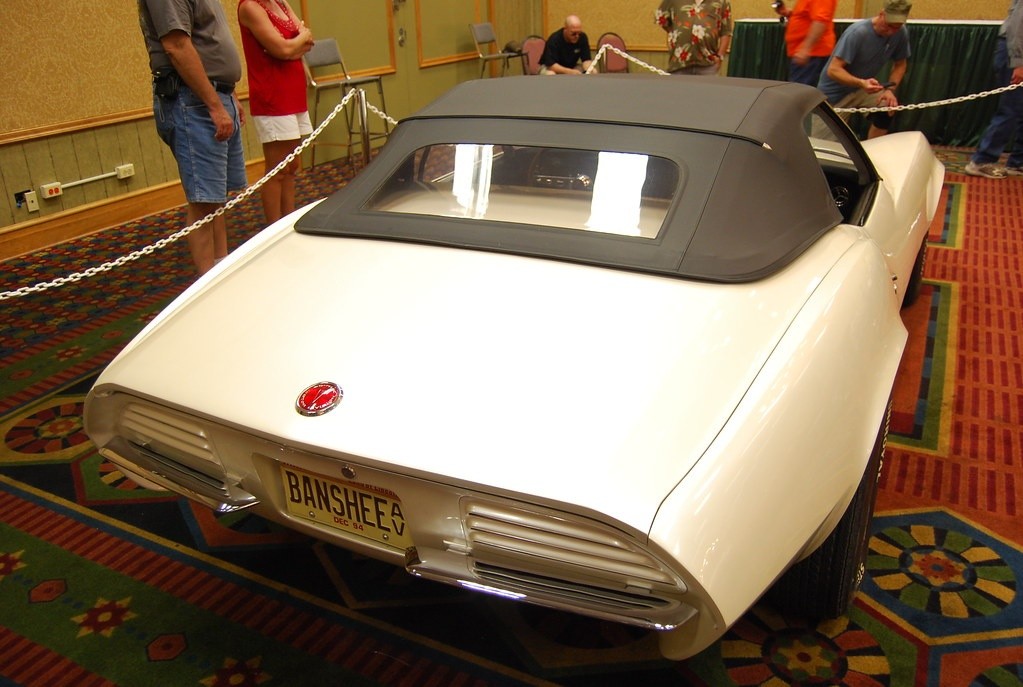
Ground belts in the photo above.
[210,80,235,95]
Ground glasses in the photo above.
[570,32,583,37]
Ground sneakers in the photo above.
[1003,164,1023,176]
[965,161,1009,179]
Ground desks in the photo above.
[726,18,1006,149]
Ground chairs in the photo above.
[520,35,547,76]
[300,39,390,172]
[596,32,629,74]
[470,22,529,79]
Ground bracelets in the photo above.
[888,87,897,96]
[716,54,724,61]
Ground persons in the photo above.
[137,0,248,278]
[537,16,596,75]
[238,0,316,224]
[653,0,733,76]
[773,1,837,86]
[966,0,1023,179]
[812,0,912,142]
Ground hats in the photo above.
[881,0,912,23]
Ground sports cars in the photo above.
[81,72,948,662]
